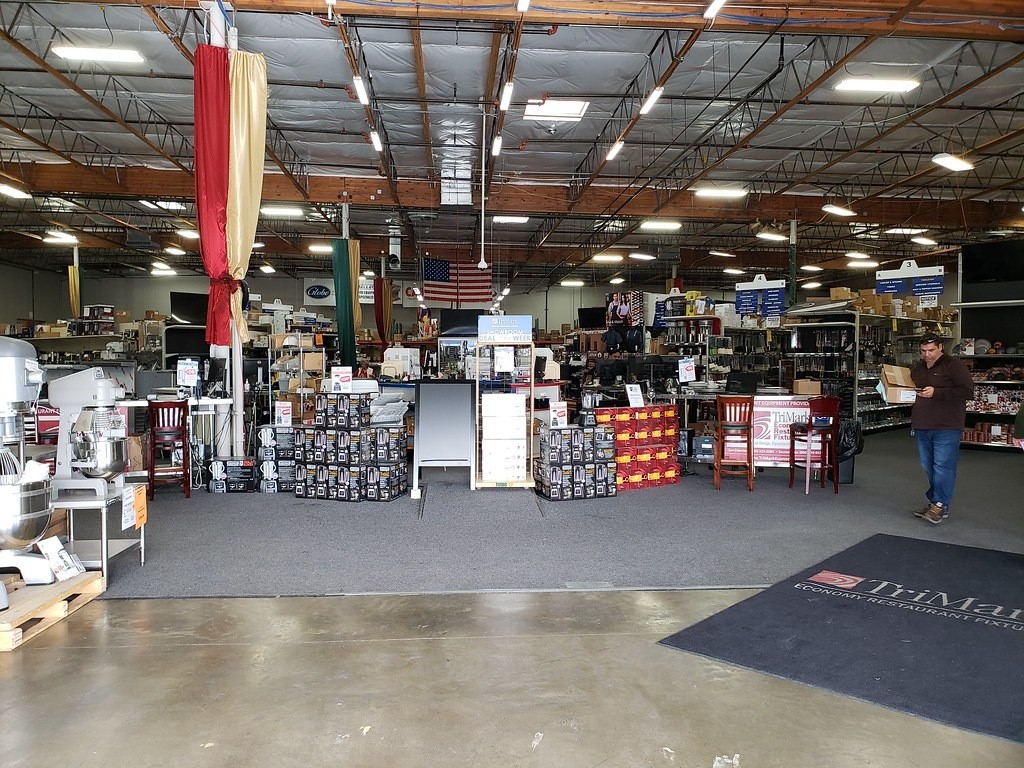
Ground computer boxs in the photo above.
[678,428,696,457]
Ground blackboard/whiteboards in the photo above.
[414,378,477,466]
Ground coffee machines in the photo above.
[661,319,714,346]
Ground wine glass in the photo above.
[647,387,656,406]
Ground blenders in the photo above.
[208,461,226,494]
[580,390,598,426]
[258,427,278,493]
[531,401,618,501]
[48,368,126,500]
[293,372,407,501]
[0,335,55,585]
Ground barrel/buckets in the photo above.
[974,339,991,353]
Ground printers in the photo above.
[692,436,714,459]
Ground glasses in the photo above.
[918,337,941,343]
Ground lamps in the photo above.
[755,226,790,243]
[931,136,976,172]
[821,190,858,217]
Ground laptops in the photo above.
[724,371,758,396]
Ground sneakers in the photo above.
[923,503,948,523]
[914,504,934,517]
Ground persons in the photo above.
[743,313,750,320]
[607,350,622,360]
[757,304,762,315]
[607,293,632,326]
[601,327,667,354]
[881,332,974,524]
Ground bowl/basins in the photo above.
[0,474,54,550]
[75,441,129,482]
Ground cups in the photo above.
[663,334,676,345]
[687,333,704,343]
[678,334,684,341]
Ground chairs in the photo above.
[713,394,755,492]
[789,395,843,496]
[148,399,191,501]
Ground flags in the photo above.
[421,257,492,302]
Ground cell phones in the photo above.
[913,387,924,394]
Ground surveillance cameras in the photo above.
[477,261,488,271]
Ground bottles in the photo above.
[40,344,93,364]
[4,320,31,338]
[909,425,915,438]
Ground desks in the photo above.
[116,397,233,477]
[643,392,831,481]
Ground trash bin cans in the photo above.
[828,419,859,482]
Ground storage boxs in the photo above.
[532,323,571,341]
[880,364,916,404]
[663,290,828,330]
[383,347,420,381]
[533,403,682,503]
[394,324,419,341]
[829,286,953,321]
[82,303,114,334]
[204,394,410,503]
[578,333,606,352]
[482,393,527,481]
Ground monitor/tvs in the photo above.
[242,358,276,391]
[627,354,690,393]
[169,290,209,325]
[534,356,547,378]
[439,309,484,336]
[595,358,627,385]
[577,306,606,328]
[208,359,220,382]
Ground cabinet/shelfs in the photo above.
[243,318,383,391]
[19,334,139,401]
[652,316,962,432]
[50,472,149,589]
[947,338,1024,453]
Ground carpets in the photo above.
[656,532,1024,744]
